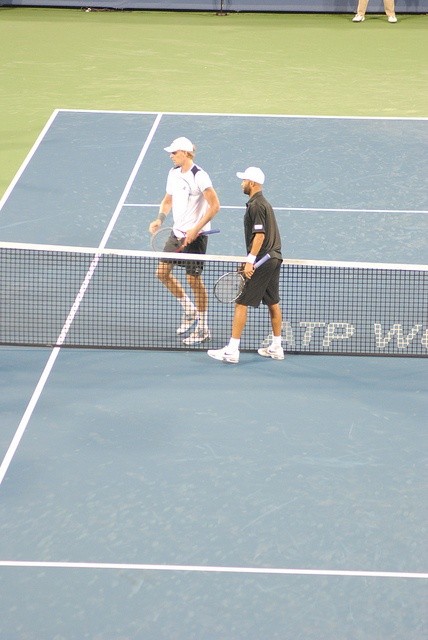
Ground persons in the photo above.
[149,136,221,346]
[352,0,398,23]
[206,166,286,363]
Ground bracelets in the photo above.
[246,253,256,265]
[157,212,166,224]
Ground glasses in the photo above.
[172,152,177,154]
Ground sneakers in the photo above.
[177,307,199,335]
[352,14,364,22]
[388,16,397,23]
[208,345,240,363]
[258,346,284,359]
[183,328,211,346]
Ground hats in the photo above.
[164,137,193,154]
[236,166,265,185]
